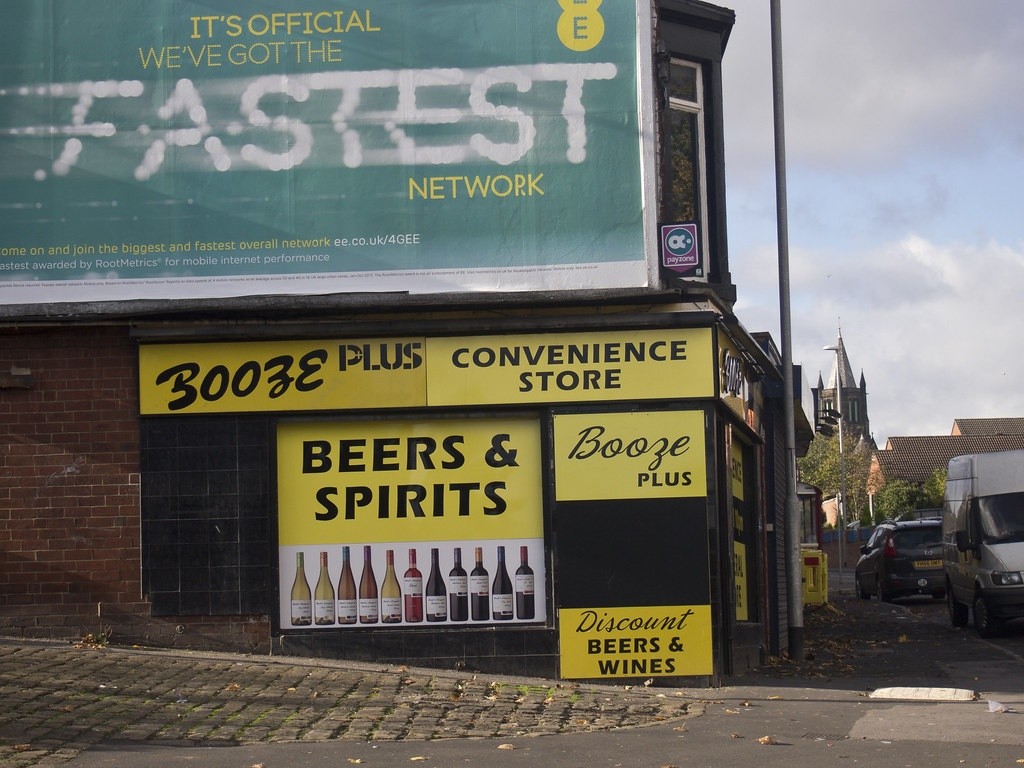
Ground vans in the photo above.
[942,449,1024,638]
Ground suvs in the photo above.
[855,517,942,603]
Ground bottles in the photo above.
[290,546,535,626]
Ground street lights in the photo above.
[822,344,848,567]
